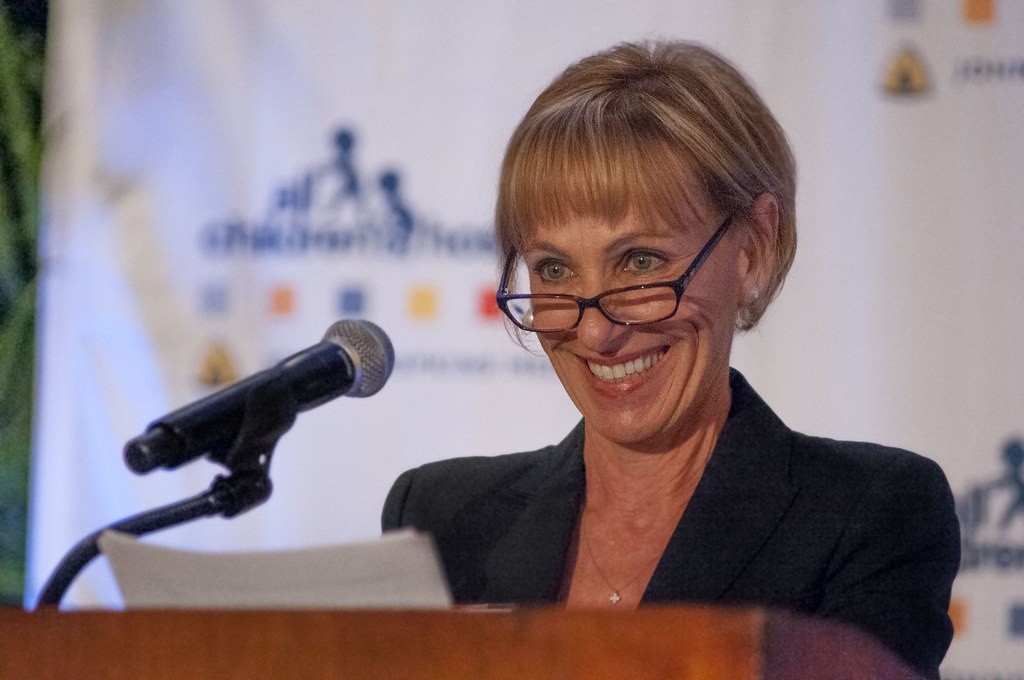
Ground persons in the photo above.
[383,36,963,680]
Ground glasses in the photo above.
[495,213,738,335]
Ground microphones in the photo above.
[122,319,395,475]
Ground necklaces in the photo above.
[582,515,662,605]
